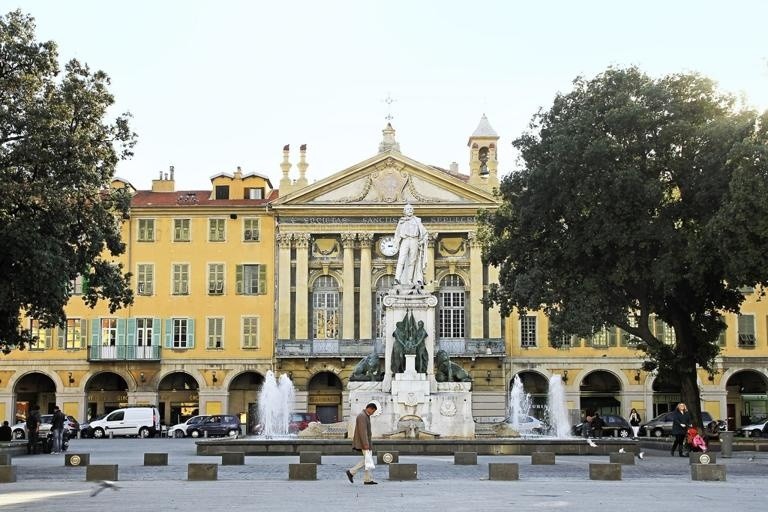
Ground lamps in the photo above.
[140,372,146,382]
[635,370,640,380]
[211,371,218,382]
[562,371,568,381]
[486,370,491,381]
[288,371,293,380]
[68,372,74,383]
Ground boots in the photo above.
[671,441,678,456]
[679,444,686,457]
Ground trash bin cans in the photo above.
[717,431,734,458]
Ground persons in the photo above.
[49,407,64,456]
[346,403,378,484]
[392,321,429,373]
[0,420,12,441]
[589,413,605,438]
[692,433,708,454]
[392,206,430,288]
[670,402,692,457]
[583,416,593,440]
[629,408,641,437]
[26,405,41,455]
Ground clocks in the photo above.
[379,235,400,257]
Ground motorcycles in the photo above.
[717,419,728,432]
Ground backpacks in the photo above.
[27,412,39,431]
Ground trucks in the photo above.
[87,407,161,438]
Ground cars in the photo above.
[500,414,544,436]
[739,416,768,437]
[187,415,242,438]
[168,415,214,438]
[571,414,634,437]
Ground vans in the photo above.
[254,412,319,435]
[639,411,716,436]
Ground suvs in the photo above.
[10,413,80,440]
[79,413,109,438]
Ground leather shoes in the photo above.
[347,470,354,483]
[364,481,378,484]
